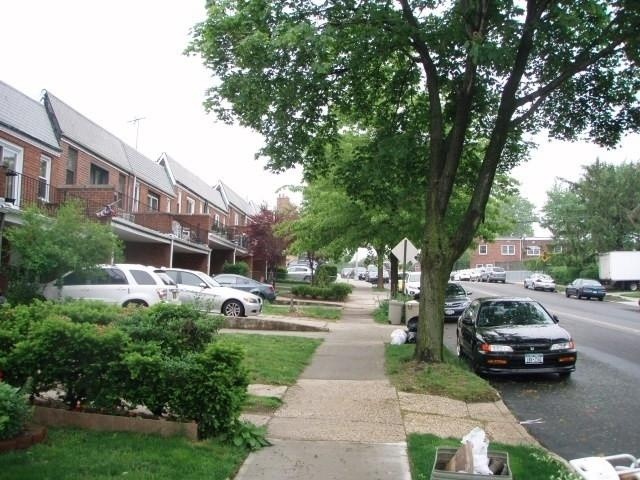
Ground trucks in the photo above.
[598,250,640,292]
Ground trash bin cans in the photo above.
[388,300,404,324]
[405,300,419,326]
[429,448,512,480]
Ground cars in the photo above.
[444,283,471,322]
[340,264,422,298]
[456,295,578,380]
[287,265,316,284]
[524,273,556,292]
[565,277,607,301]
[21,263,277,317]
[451,267,507,283]
